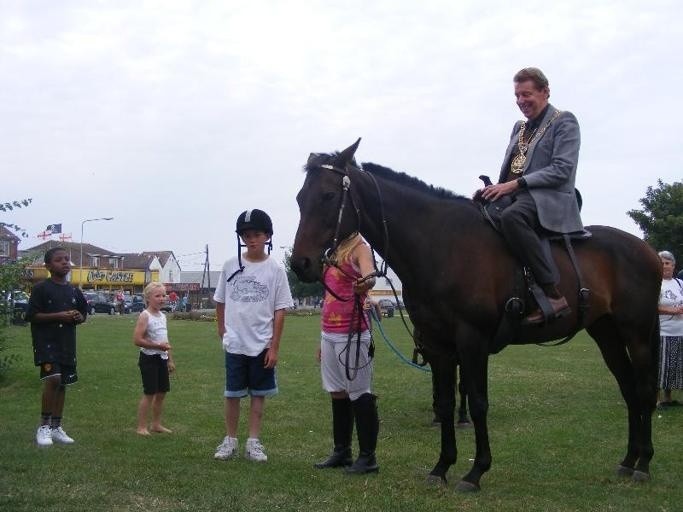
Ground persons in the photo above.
[91,290,192,313]
[133,281,176,436]
[316,230,383,474]
[475,63,587,327]
[212,207,294,465]
[26,247,89,448]
[654,250,682,406]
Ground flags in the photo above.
[47,222,63,234]
[37,230,51,240]
[59,233,72,241]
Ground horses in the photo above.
[285,133,667,495]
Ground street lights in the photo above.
[79,215,115,295]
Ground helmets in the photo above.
[236,210,273,234]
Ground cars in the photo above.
[0,290,191,319]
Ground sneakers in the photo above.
[215,436,238,459]
[51,427,75,443]
[245,439,267,461]
[37,425,53,445]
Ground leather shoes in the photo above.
[522,295,571,326]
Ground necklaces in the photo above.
[511,111,561,178]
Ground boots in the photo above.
[313,398,354,468]
[345,394,379,475]
